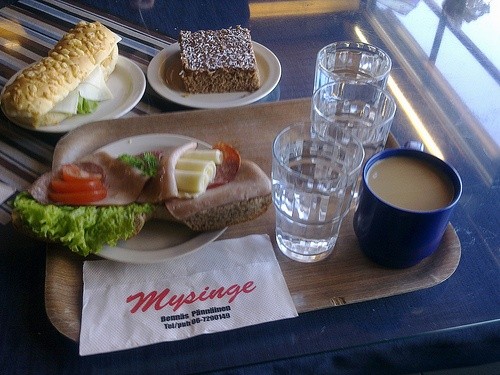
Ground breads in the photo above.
[150,193,273,232]
[178,24,260,95]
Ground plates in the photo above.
[0,55,146,132]
[147,40,282,110]
[85,133,229,264]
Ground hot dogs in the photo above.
[1,20,122,128]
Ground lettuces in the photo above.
[11,151,159,257]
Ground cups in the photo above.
[314,42,391,94]
[310,81,396,162]
[353,140,463,272]
[272,121,365,264]
